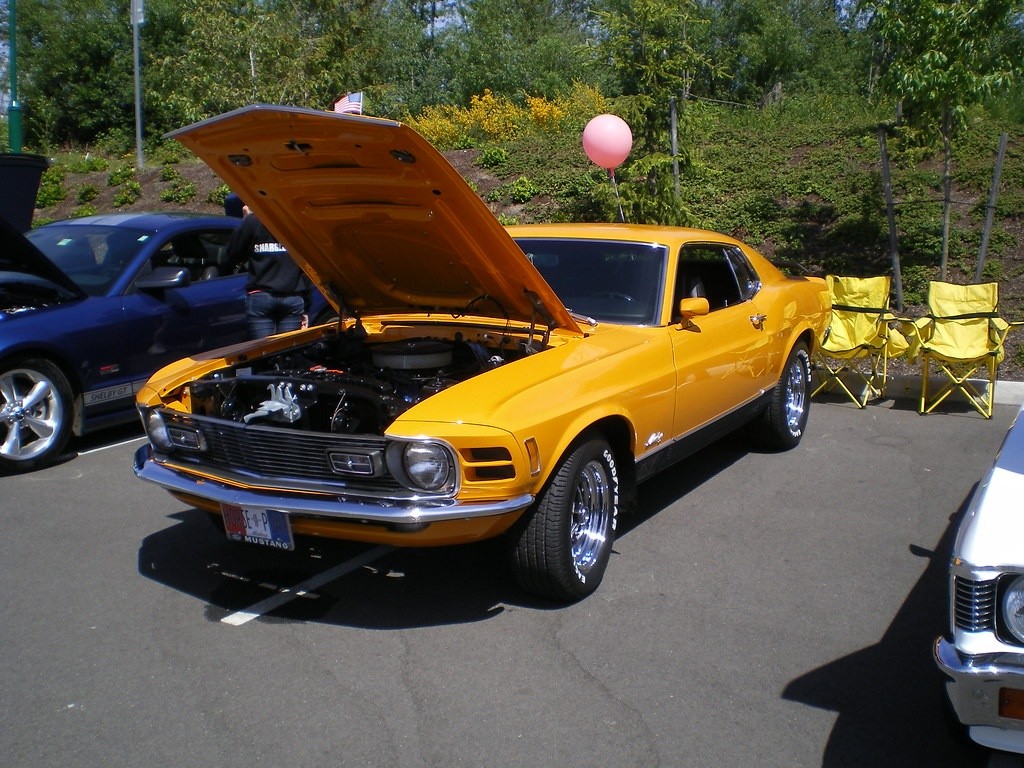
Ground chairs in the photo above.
[105,232,152,280]
[645,270,706,318]
[898,281,1024,421]
[173,239,219,282]
[810,274,914,411]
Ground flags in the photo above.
[335,93,360,113]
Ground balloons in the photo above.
[582,114,631,168]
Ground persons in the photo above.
[222,194,340,340]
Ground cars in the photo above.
[0,150,331,475]
[130,104,833,609]
[933,399,1024,759]
